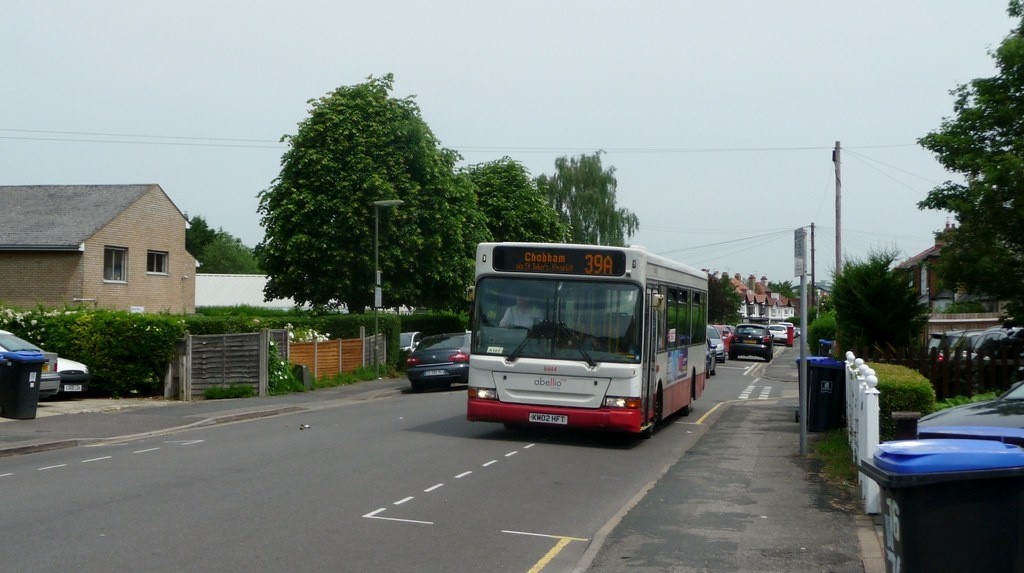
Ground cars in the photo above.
[793,327,798,338]
[407,332,471,388]
[728,324,775,363]
[399,331,424,353]
[916,377,1024,430]
[726,325,735,335]
[767,324,787,343]
[706,337,717,379]
[713,325,732,352]
[797,328,800,336]
[56,357,89,394]
[0,330,59,395]
[928,326,1024,362]
[707,325,727,364]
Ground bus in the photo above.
[463,241,708,432]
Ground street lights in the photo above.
[375,198,404,309]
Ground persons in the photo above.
[498,289,545,331]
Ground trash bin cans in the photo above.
[855,439,1023,573]
[0,350,44,419]
[807,363,846,432]
[795,357,830,422]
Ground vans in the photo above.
[778,322,793,328]
